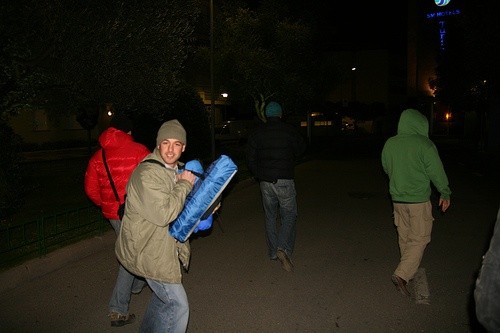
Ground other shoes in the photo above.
[414,295,432,307]
[391,273,410,297]
[277,248,295,273]
[108,311,136,327]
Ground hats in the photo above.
[157,119,187,145]
[264,102,283,119]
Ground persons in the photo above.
[254,102,303,273]
[84,116,222,333]
[382,108,452,305]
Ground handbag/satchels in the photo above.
[117,203,124,221]
